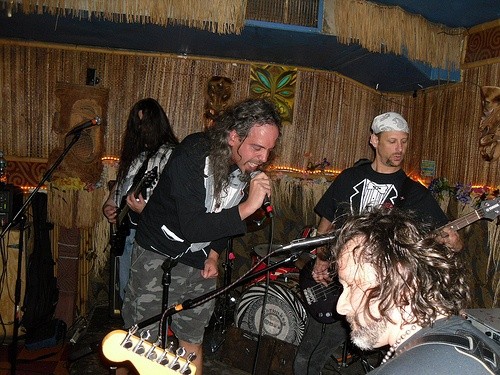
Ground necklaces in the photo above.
[381,309,445,364]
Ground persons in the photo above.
[293,112,462,375]
[332,204,500,375]
[115,95,283,375]
[102,98,180,300]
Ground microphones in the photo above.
[250,171,273,219]
[274,231,338,251]
[66,116,102,136]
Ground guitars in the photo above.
[110,166,158,257]
[299,195,500,324]
[101,323,197,375]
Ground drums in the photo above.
[232,278,309,345]
[293,224,318,269]
[244,242,300,290]
